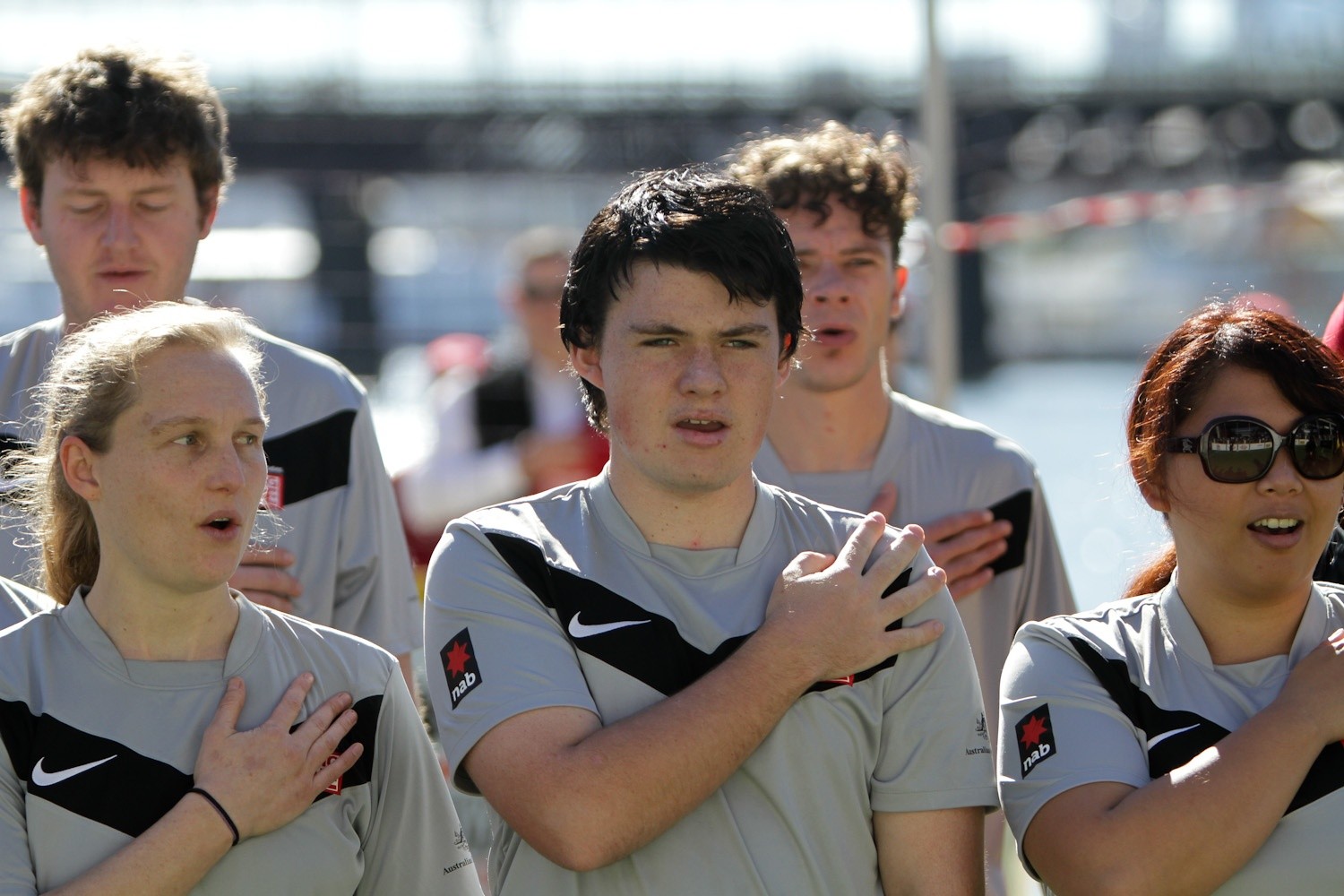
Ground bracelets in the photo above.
[185,787,239,850]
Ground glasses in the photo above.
[1165,416,1344,484]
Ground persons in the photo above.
[2,301,497,895]
[996,291,1344,895]
[2,41,435,744]
[419,157,1010,895]
[686,111,1089,896]
[400,219,605,544]
